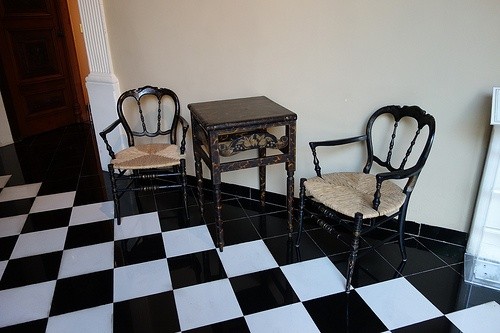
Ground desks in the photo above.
[187,96,296,251]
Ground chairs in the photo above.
[298,105,435,295]
[99,86,189,226]
